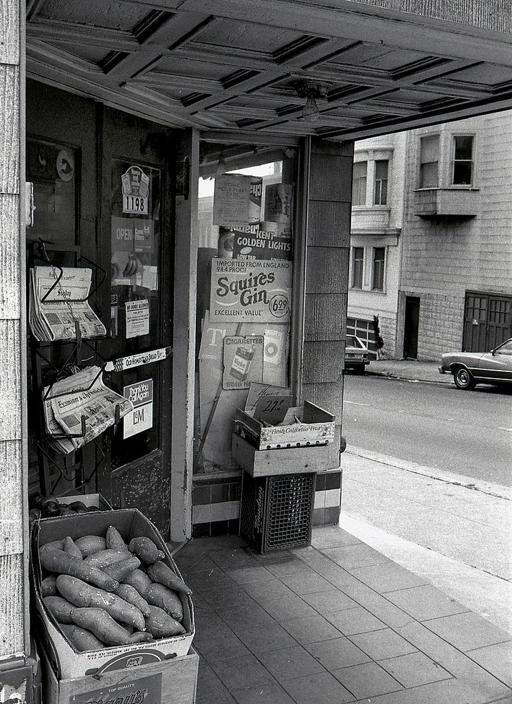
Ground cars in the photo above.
[345,335,371,374]
[440,334,512,392]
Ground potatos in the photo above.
[38,524,194,651]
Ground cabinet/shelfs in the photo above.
[27,238,107,499]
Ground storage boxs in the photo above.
[29,508,200,704]
[232,399,335,478]
